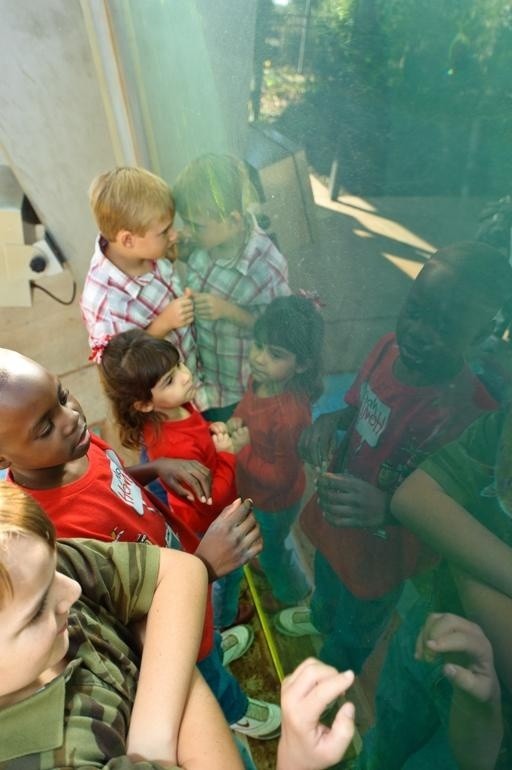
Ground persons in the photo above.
[97,327,257,633]
[227,295,325,617]
[79,165,208,505]
[0,344,281,744]
[411,610,506,770]
[355,401,511,770]
[269,238,512,678]
[274,655,358,770]
[0,479,244,770]
[172,148,294,421]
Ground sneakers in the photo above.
[229,696,284,741]
[218,624,255,668]
[272,605,322,638]
